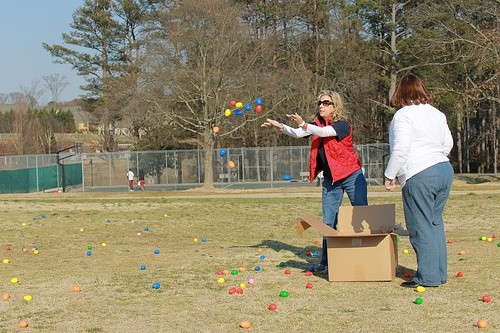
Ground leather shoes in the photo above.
[401,281,424,288]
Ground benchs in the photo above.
[299,172,310,182]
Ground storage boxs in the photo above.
[296,204,410,282]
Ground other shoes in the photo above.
[313,264,328,275]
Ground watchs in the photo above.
[298,121,305,128]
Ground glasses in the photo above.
[318,100,334,105]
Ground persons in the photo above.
[383,75,454,288]
[261,90,368,273]
[126,168,145,192]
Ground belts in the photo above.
[323,172,332,176]
